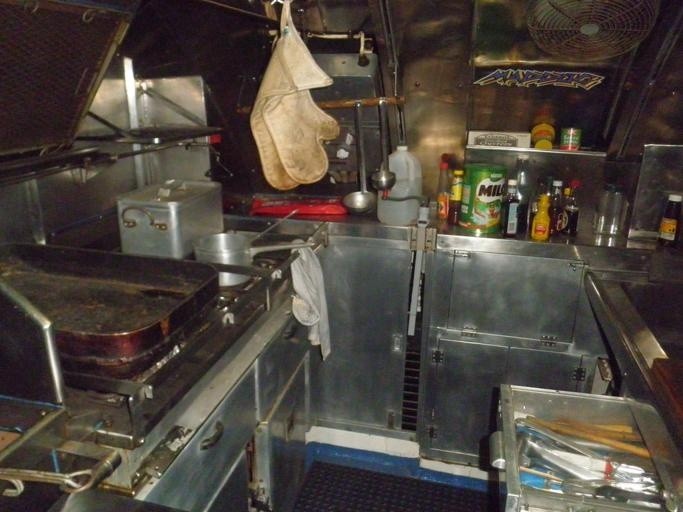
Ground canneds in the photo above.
[559,127,582,153]
[457,162,506,235]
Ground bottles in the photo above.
[590,182,628,237]
[438,153,579,240]
[656,195,682,247]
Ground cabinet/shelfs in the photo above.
[144,308,319,511]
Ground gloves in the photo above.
[246,21,342,193]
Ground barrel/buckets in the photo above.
[375,144,423,228]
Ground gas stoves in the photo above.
[0,228,318,448]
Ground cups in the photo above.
[530,124,556,148]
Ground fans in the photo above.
[525,1,662,62]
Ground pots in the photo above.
[193,232,313,287]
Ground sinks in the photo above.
[601,274,683,359]
